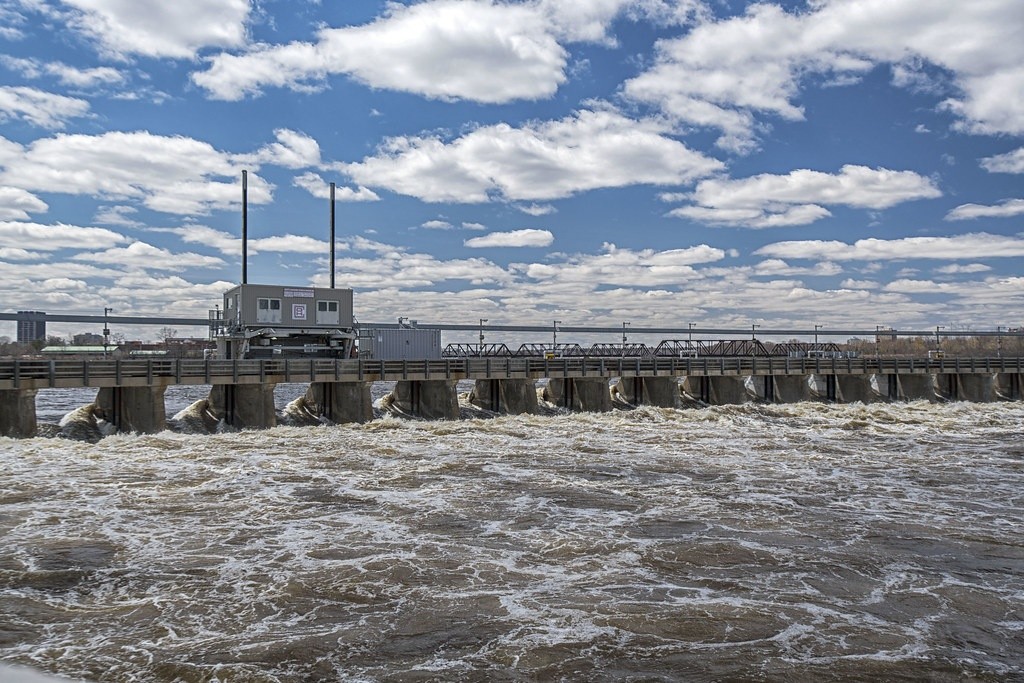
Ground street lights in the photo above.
[623,321,630,358]
[998,326,1005,357]
[815,324,823,358]
[753,324,760,357]
[876,325,884,361]
[553,321,561,359]
[104,308,112,356]
[480,319,488,358]
[689,323,696,357]
[936,326,945,357]
